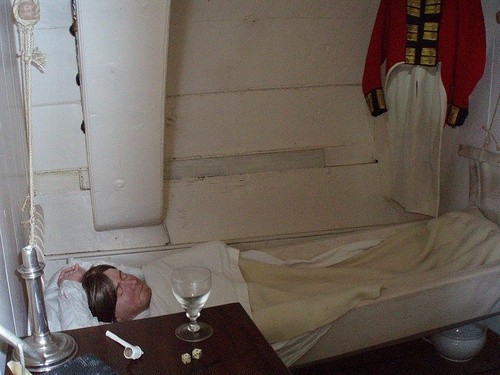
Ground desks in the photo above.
[4,302,294,375]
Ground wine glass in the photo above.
[170,266,213,342]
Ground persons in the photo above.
[57,263,151,323]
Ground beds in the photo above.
[28,210,500,367]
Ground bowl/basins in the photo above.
[432,318,487,363]
